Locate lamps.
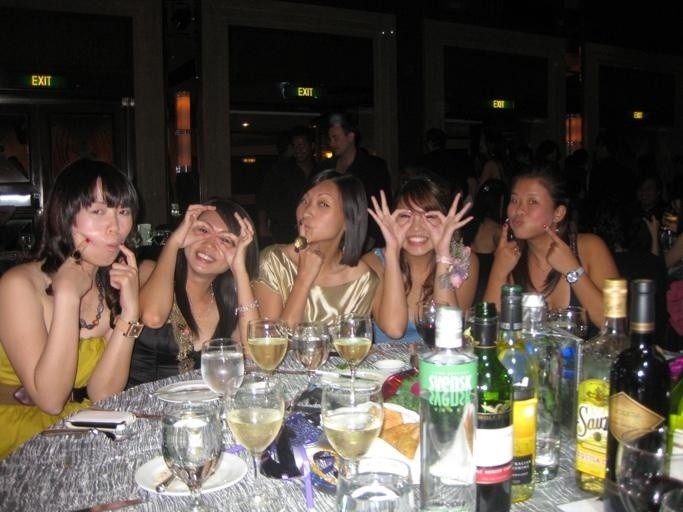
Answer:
[564,111,584,158]
[170,87,195,176]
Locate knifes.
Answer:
[67,498,144,511]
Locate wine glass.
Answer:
[200,312,377,405]
[158,376,415,512]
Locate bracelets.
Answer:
[234,299,260,316]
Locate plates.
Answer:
[372,359,405,374]
[155,379,226,405]
[135,452,248,497]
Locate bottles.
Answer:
[415,276,683,512]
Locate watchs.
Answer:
[112,316,143,339]
[564,268,586,288]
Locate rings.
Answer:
[293,236,306,253]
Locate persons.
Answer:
[252,166,380,341]
[125,195,261,391]
[481,166,625,340]
[359,173,481,342]
[0,158,141,461]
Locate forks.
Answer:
[38,429,144,442]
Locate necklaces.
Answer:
[166,284,216,325]
[78,272,105,331]
